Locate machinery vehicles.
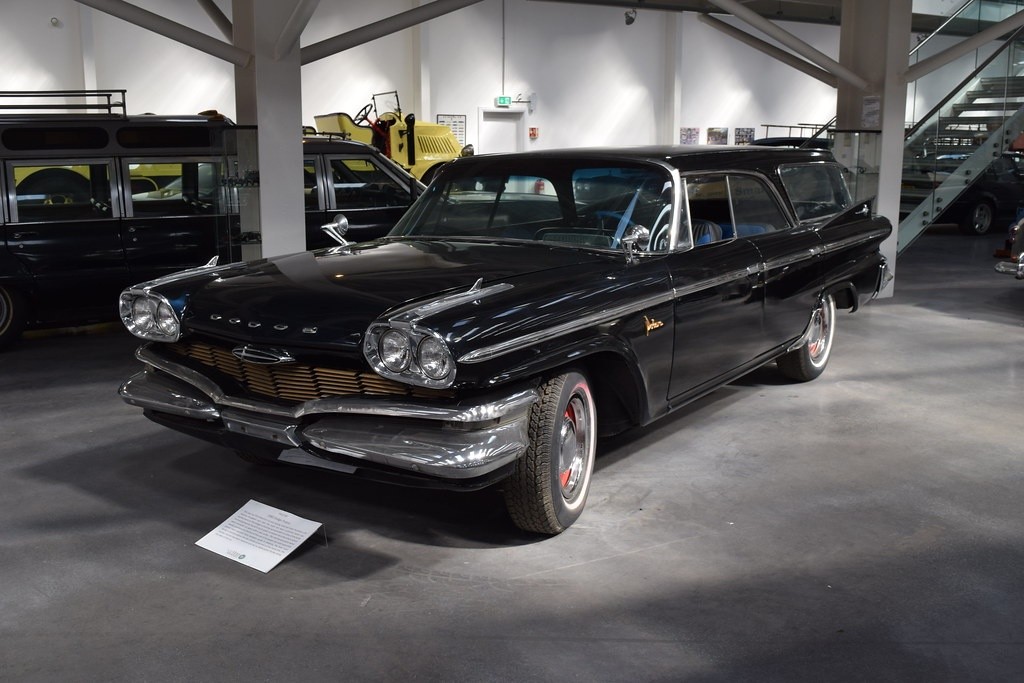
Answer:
[15,92,463,202]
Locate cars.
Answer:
[119,138,893,533]
[910,149,1024,237]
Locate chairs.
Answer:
[657,218,723,248]
[723,222,775,240]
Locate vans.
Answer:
[0,90,602,348]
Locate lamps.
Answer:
[624,8,638,25]
